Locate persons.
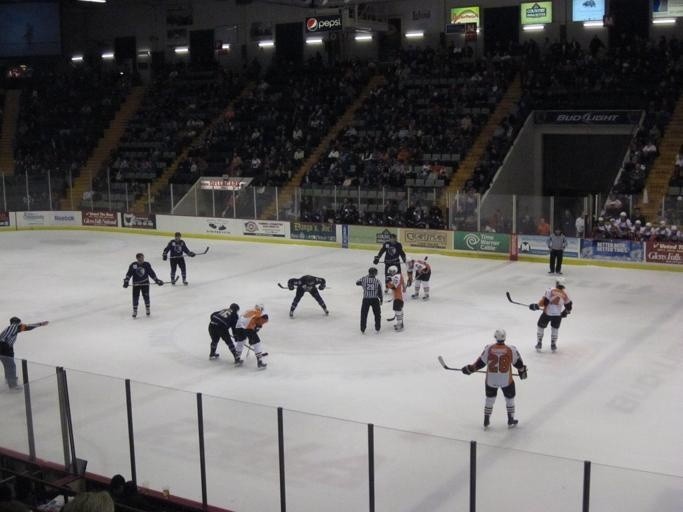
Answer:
[234,304,268,367]
[164,232,198,284]
[2,33,681,241]
[121,253,164,317]
[407,259,431,301]
[110,475,126,502]
[530,278,573,351]
[208,304,240,361]
[287,273,329,316]
[0,317,50,392]
[355,267,383,331]
[386,266,404,329]
[547,226,569,274]
[373,234,408,292]
[124,481,137,506]
[462,328,527,425]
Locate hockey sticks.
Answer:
[126,277,180,284]
[506,291,542,309]
[374,256,428,264]
[161,246,209,259]
[278,280,319,291]
[386,316,398,321]
[230,333,267,356]
[245,342,251,359]
[437,356,524,376]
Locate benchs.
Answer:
[1,457,147,512]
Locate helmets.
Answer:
[305,234,413,285]
[556,276,565,286]
[136,232,265,311]
[494,328,506,342]
[10,317,20,324]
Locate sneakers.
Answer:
[289,289,429,332]
[484,415,489,426]
[536,342,541,348]
[551,344,556,350]
[210,352,267,368]
[133,310,137,316]
[509,414,518,424]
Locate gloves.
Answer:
[518,365,528,379]
[561,311,567,317]
[123,281,129,287]
[462,364,475,374]
[530,304,539,311]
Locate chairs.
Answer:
[519,48,682,235]
